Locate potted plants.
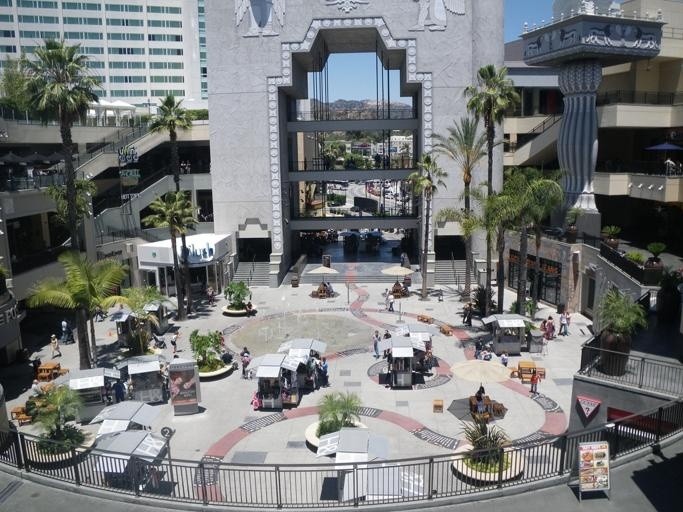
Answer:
[565,206,584,243]
[596,284,649,376]
[655,264,682,328]
[601,224,621,251]
[644,241,667,268]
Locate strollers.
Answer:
[479,345,491,360]
[152,331,167,350]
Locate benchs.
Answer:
[415,314,434,326]
[439,324,452,336]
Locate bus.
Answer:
[327,181,349,191]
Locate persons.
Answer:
[372,329,380,359]
[663,158,676,172]
[321,357,328,387]
[384,348,391,370]
[378,205,385,217]
[387,292,394,311]
[475,395,486,412]
[170,331,182,353]
[30,379,49,399]
[303,157,308,171]
[391,281,408,299]
[245,300,255,317]
[50,333,61,359]
[318,281,332,299]
[215,328,251,380]
[24,395,39,423]
[60,317,75,345]
[462,303,468,324]
[374,152,389,170]
[186,160,191,174]
[382,329,391,359]
[502,351,507,366]
[179,160,186,174]
[94,305,104,321]
[473,336,492,361]
[200,209,213,221]
[383,288,389,309]
[159,361,169,390]
[466,303,473,325]
[170,373,183,398]
[180,374,195,390]
[111,377,125,403]
[474,385,486,397]
[528,370,541,395]
[203,284,215,305]
[539,309,571,341]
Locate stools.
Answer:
[470,394,505,423]
[507,361,546,384]
[433,399,443,413]
[9,361,69,427]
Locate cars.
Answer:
[364,178,405,200]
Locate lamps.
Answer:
[627,182,663,193]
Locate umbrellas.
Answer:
[305,265,339,282]
[84,94,136,117]
[380,264,414,282]
[0,152,75,166]
[644,141,682,159]
